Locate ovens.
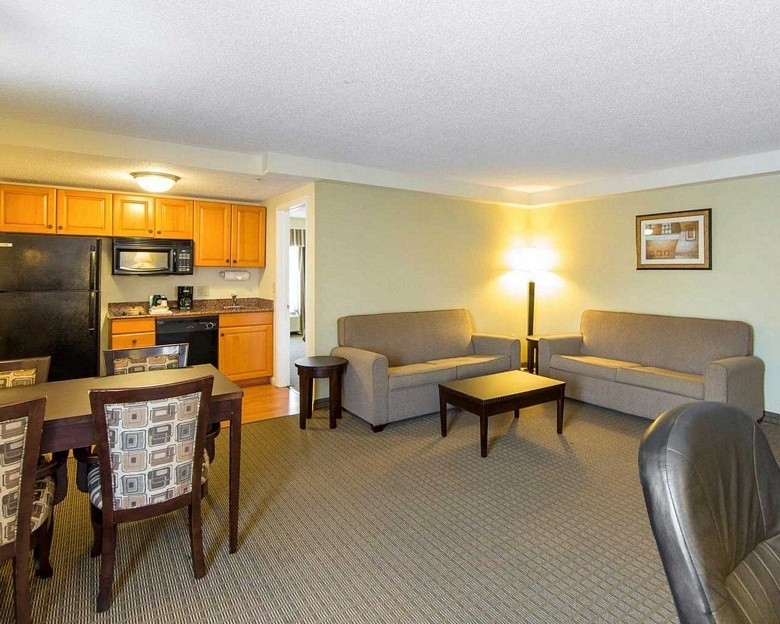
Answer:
[154,315,219,370]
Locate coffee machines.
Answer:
[177,286,193,309]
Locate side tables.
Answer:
[295,356,349,430]
[524,333,549,377]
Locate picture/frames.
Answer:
[635,207,713,271]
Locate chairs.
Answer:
[0,356,69,624]
[637,399,780,624]
[73,343,208,614]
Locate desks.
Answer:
[0,365,245,554]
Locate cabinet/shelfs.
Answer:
[0,184,112,237]
[111,317,155,349]
[113,194,193,239]
[219,312,272,379]
[195,201,266,267]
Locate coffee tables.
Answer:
[438,370,567,456]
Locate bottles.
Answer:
[160,296,168,306]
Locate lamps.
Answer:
[129,171,181,194]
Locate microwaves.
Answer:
[112,237,194,275]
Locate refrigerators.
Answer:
[0,232,102,383]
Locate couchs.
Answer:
[540,309,765,422]
[329,308,522,433]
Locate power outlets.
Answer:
[197,286,210,296]
